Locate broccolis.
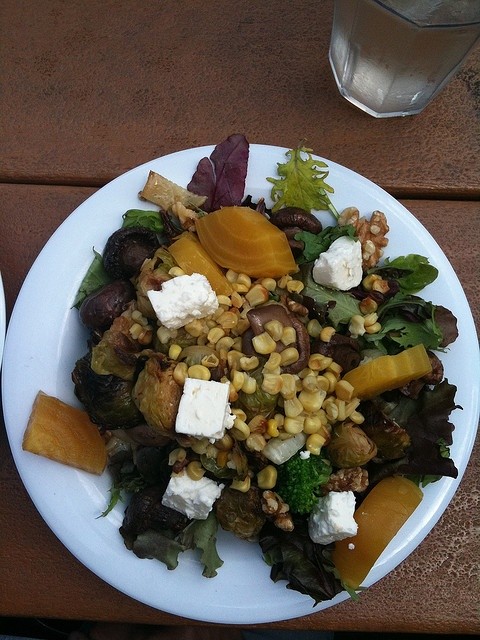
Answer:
[279,454,332,515]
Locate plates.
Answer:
[2,143,480,624]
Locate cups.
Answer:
[329,0,480,119]
